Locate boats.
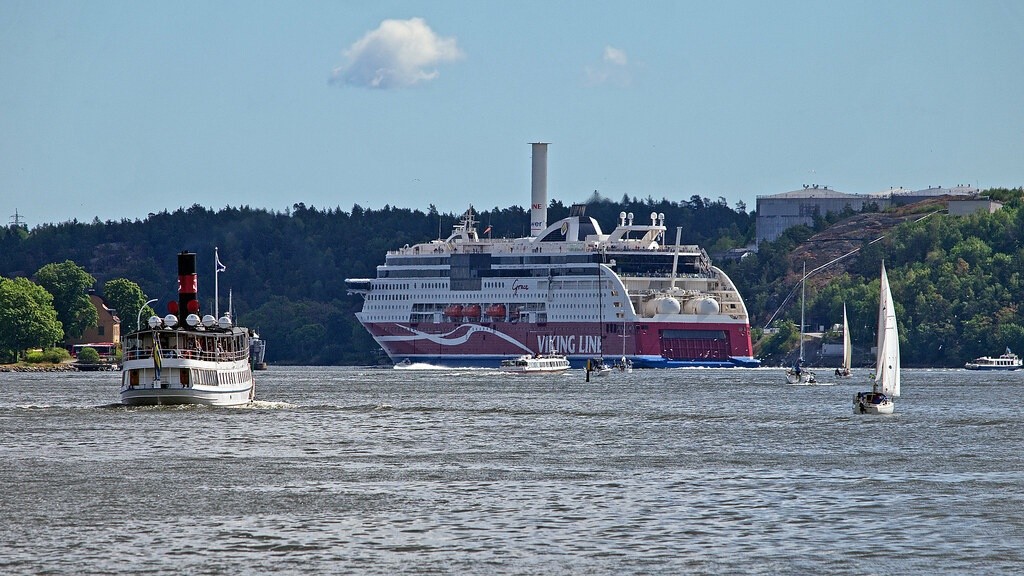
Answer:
[499,342,572,373]
[442,306,462,317]
[460,306,482,317]
[964,346,1023,371]
[121,247,257,406]
[345,143,761,370]
[210,301,267,371]
[485,306,505,316]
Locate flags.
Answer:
[150,329,162,381]
[215,251,226,272]
[483,228,491,234]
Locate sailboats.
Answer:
[785,261,816,384]
[835,302,854,379]
[853,259,900,414]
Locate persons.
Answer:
[129,338,222,361]
[842,369,849,376]
[534,353,540,359]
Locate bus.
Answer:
[72,342,116,358]
